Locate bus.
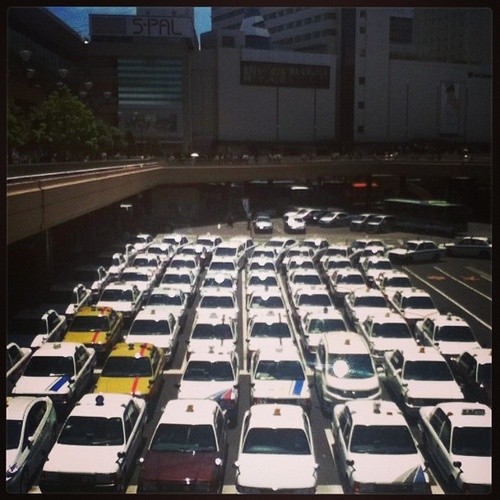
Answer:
[312,178,380,211]
[382,197,468,236]
[246,181,311,205]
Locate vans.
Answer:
[283,205,397,234]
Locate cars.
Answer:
[281,237,492,404]
[38,392,147,493]
[388,240,446,264]
[255,213,272,232]
[6,232,222,401]
[234,403,318,493]
[136,398,229,492]
[6,396,58,490]
[440,238,492,260]
[331,397,426,493]
[176,232,254,400]
[419,401,492,490]
[247,236,316,415]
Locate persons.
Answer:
[242,152,248,160]
[168,152,175,160]
[225,212,233,229]
[268,151,281,163]
